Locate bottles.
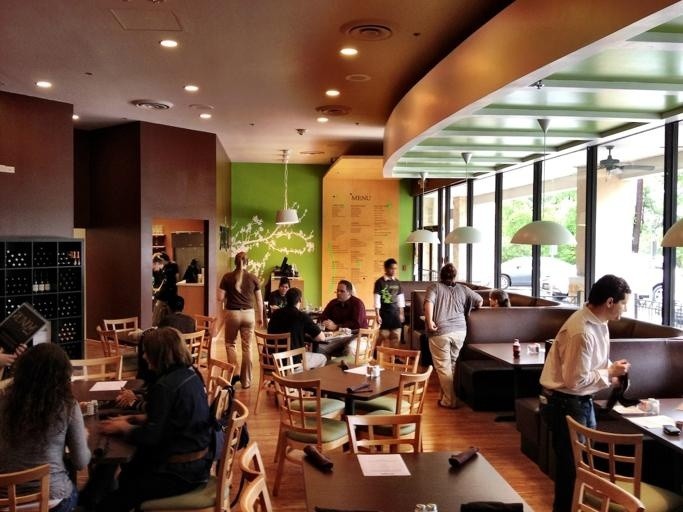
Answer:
[0,246,81,344]
[512,338,520,360]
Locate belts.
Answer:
[166,448,209,465]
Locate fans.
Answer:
[572,143,657,183]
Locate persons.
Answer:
[315,280,368,364]
[152,251,179,325]
[536,275,633,511]
[216,252,264,388]
[422,265,483,406]
[0,344,92,511]
[114,326,207,416]
[267,277,292,317]
[155,299,198,336]
[181,258,201,283]
[373,258,406,365]
[263,287,327,369]
[0,342,28,381]
[94,327,216,511]
[488,290,514,310]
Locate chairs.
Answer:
[566,412,681,511]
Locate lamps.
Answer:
[404,151,481,247]
[275,150,299,225]
[510,81,579,248]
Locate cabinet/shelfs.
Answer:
[1,234,86,356]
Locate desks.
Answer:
[300,450,534,511]
[176,280,203,315]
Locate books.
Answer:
[0,302,49,353]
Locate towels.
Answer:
[460,501,526,512]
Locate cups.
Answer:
[647,397,660,416]
[366,365,380,380]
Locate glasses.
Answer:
[337,289,346,293]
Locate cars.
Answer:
[498,255,577,292]
